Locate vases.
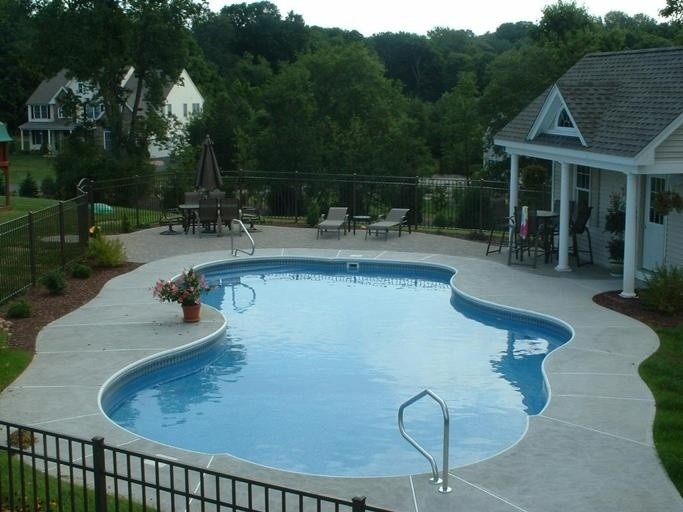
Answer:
[182,304,201,322]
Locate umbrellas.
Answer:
[192,134,222,199]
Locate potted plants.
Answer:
[606,239,625,278]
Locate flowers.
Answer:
[153,269,210,305]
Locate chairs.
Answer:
[485,199,594,269]
[315,206,349,240]
[366,207,412,240]
[155,192,264,236]
